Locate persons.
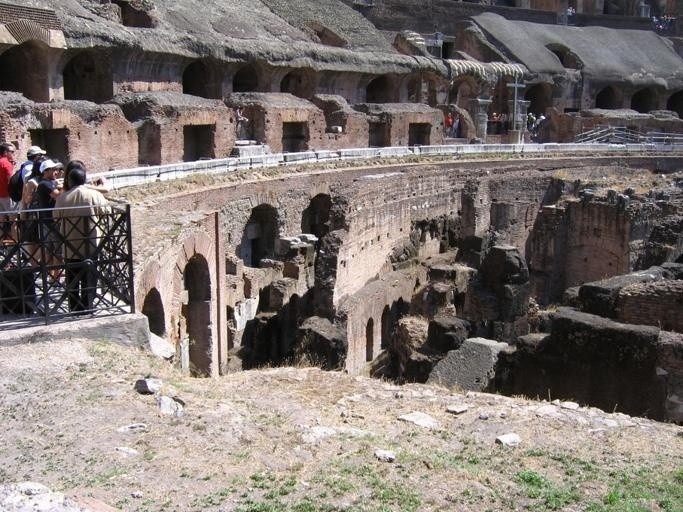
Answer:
[0,142,20,241]
[445,112,453,138]
[452,114,459,137]
[524,111,546,139]
[8,145,109,315]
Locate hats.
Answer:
[38,159,60,172]
[27,145,48,157]
